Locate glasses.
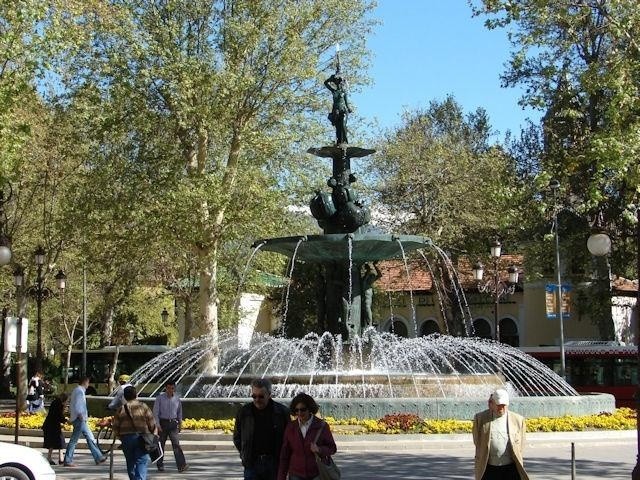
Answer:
[292,406,309,412]
[251,392,268,399]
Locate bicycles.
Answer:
[96,407,119,454]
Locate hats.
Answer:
[491,389,510,407]
[118,374,130,384]
[313,453,342,480]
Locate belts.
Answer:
[160,418,177,422]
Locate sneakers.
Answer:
[157,465,166,472]
[49,459,78,468]
[94,456,107,465]
[178,463,189,473]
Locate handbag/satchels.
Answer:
[137,432,159,454]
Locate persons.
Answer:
[25,369,190,480]
[323,73,352,144]
[471,388,530,480]
[233,377,292,479]
[277,393,337,479]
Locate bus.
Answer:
[504,339,639,413]
[59,344,201,398]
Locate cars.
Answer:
[0,439,57,479]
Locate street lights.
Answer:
[1,179,18,270]
[471,237,521,378]
[14,244,69,374]
[586,204,640,305]
